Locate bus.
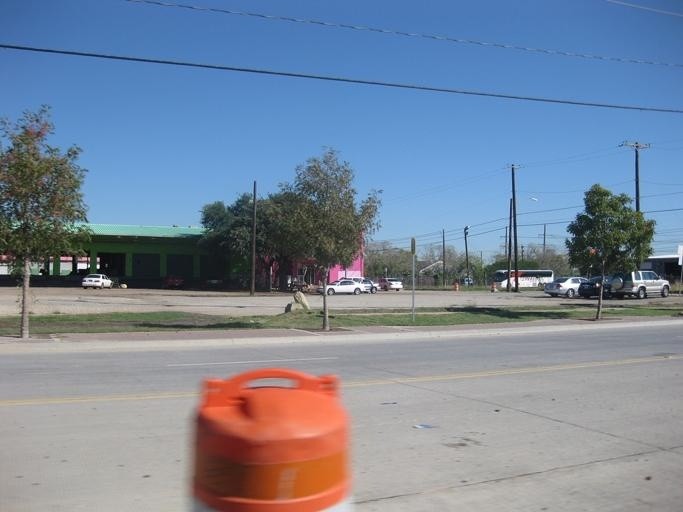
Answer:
[493,269,554,289]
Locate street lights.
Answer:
[520,245,524,263]
[505,196,539,292]
[463,225,470,288]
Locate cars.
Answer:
[356,278,376,294]
[375,278,403,291]
[339,277,364,284]
[202,275,223,291]
[287,281,309,290]
[545,277,589,299]
[81,274,113,290]
[316,280,365,295]
[578,275,626,299]
[160,275,184,290]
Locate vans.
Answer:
[452,277,473,288]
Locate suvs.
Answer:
[611,270,671,299]
